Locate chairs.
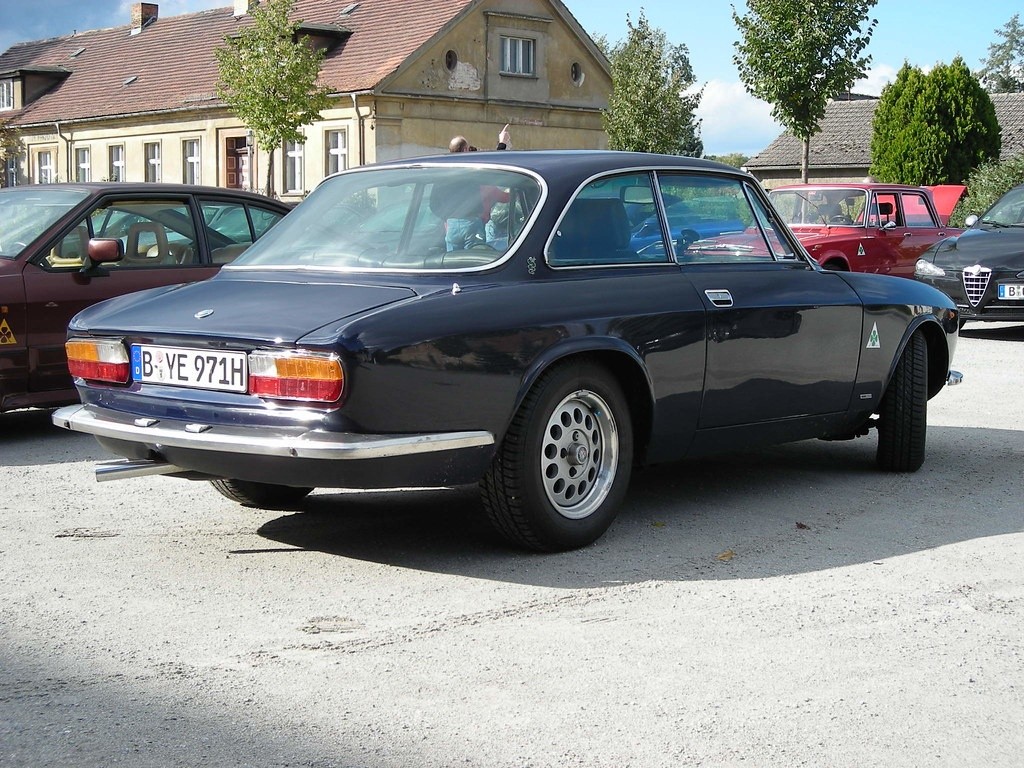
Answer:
[116,222,177,266]
[41,227,88,267]
[871,203,892,226]
[553,197,637,259]
[818,204,843,222]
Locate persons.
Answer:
[430,124,523,251]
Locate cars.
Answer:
[0,180,295,420]
[623,187,745,264]
[61,201,274,256]
[53,148,962,558]
[914,181,1024,332]
[687,181,968,284]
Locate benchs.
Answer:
[286,247,504,268]
[137,243,250,264]
